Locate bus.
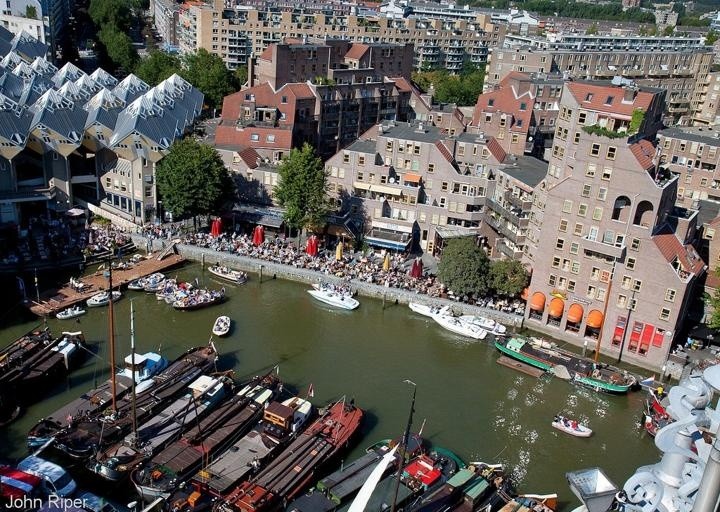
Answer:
[85,37,95,56]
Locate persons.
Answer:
[69,276,83,293]
[657,384,664,397]
[554,412,583,432]
[92,215,527,331]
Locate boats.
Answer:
[406,301,676,439]
[55,261,249,320]
[307,289,360,311]
[312,283,354,299]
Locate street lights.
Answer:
[614,289,640,365]
[157,200,163,238]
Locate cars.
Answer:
[144,9,162,42]
